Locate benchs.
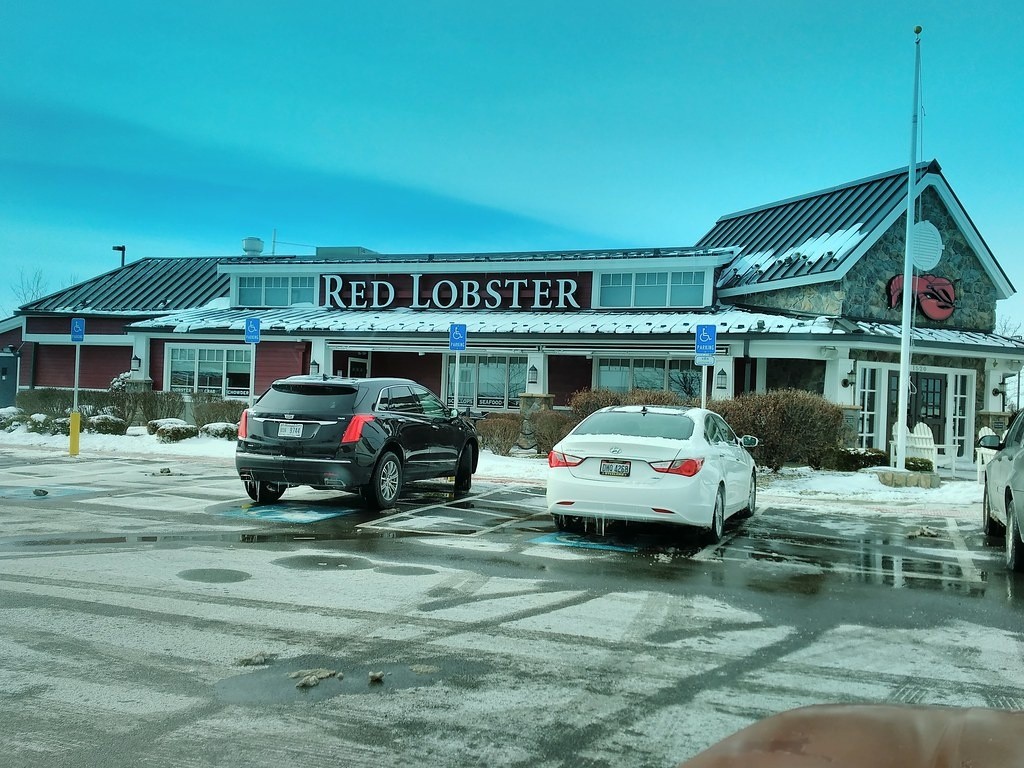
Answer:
[889,421,961,479]
[974,426,1010,483]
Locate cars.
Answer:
[235,373,479,509]
[546,405,758,543]
[977,407,1024,571]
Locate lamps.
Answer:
[130,355,142,371]
[716,368,727,389]
[310,360,319,375]
[2,346,17,353]
[528,364,538,383]
[847,370,856,386]
[993,377,1008,397]
